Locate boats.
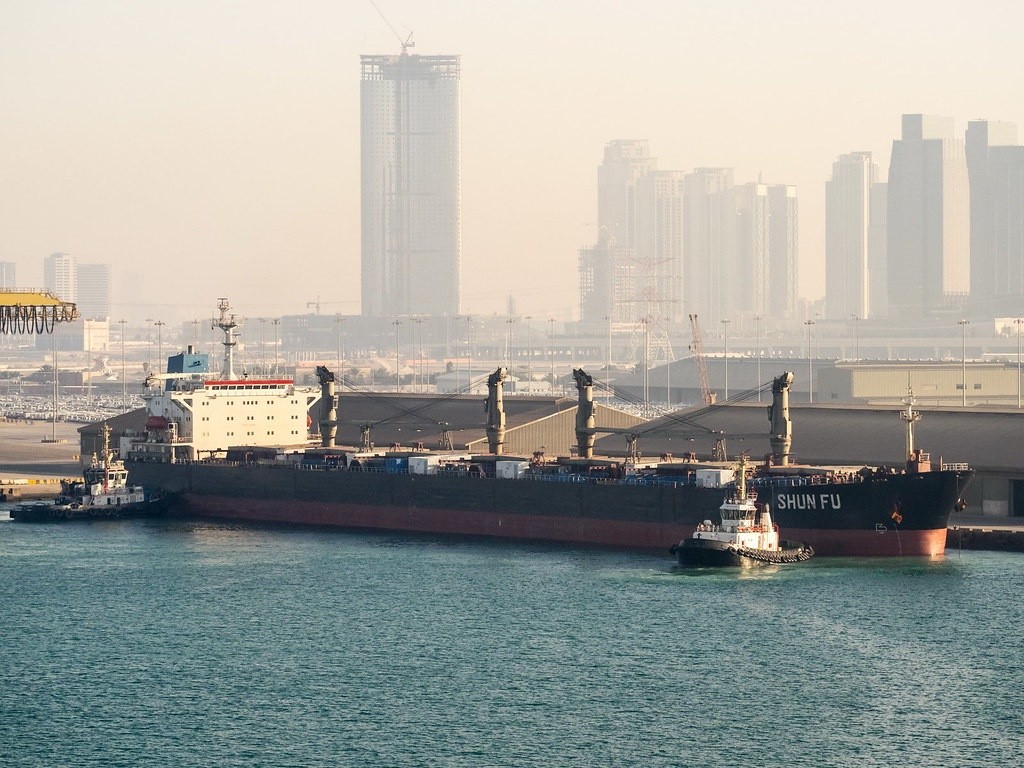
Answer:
[74,298,974,566]
[670,448,816,568]
[9,422,178,521]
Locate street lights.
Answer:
[547,318,556,397]
[849,312,861,365]
[153,320,166,395]
[146,319,153,376]
[602,317,610,391]
[119,319,127,414]
[753,314,763,403]
[410,319,417,395]
[464,317,473,394]
[416,319,425,394]
[392,320,402,394]
[639,317,652,414]
[334,317,347,391]
[524,314,534,396]
[957,320,971,406]
[663,314,673,408]
[244,316,281,374]
[191,320,200,355]
[506,318,516,396]
[803,319,816,403]
[1014,318,1024,409]
[720,318,732,401]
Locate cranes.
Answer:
[687,312,718,405]
[307,296,360,314]
